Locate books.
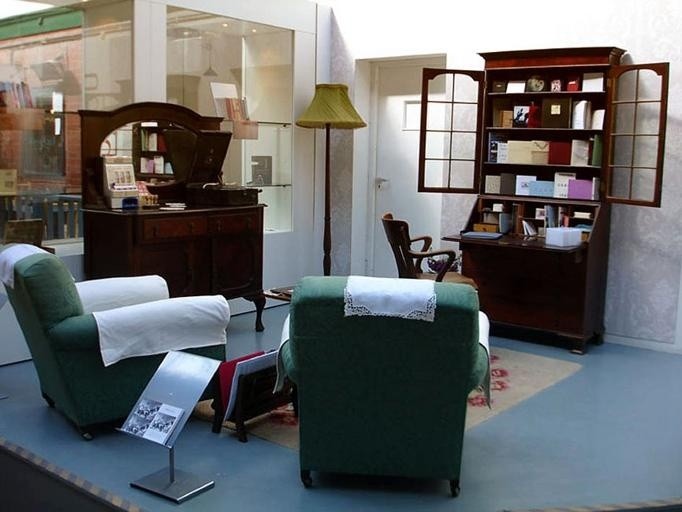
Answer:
[572,100,591,130]
[514,106,530,128]
[123,397,186,446]
[591,176,600,201]
[498,212,511,233]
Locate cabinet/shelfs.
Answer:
[216,119,295,234]
[442,45,627,354]
[78,198,268,331]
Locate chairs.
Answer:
[0,242,231,439]
[0,191,83,239]
[381,212,479,287]
[277,272,492,497]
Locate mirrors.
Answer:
[94,118,199,191]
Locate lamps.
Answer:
[296,81,368,275]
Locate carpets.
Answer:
[189,346,581,453]
